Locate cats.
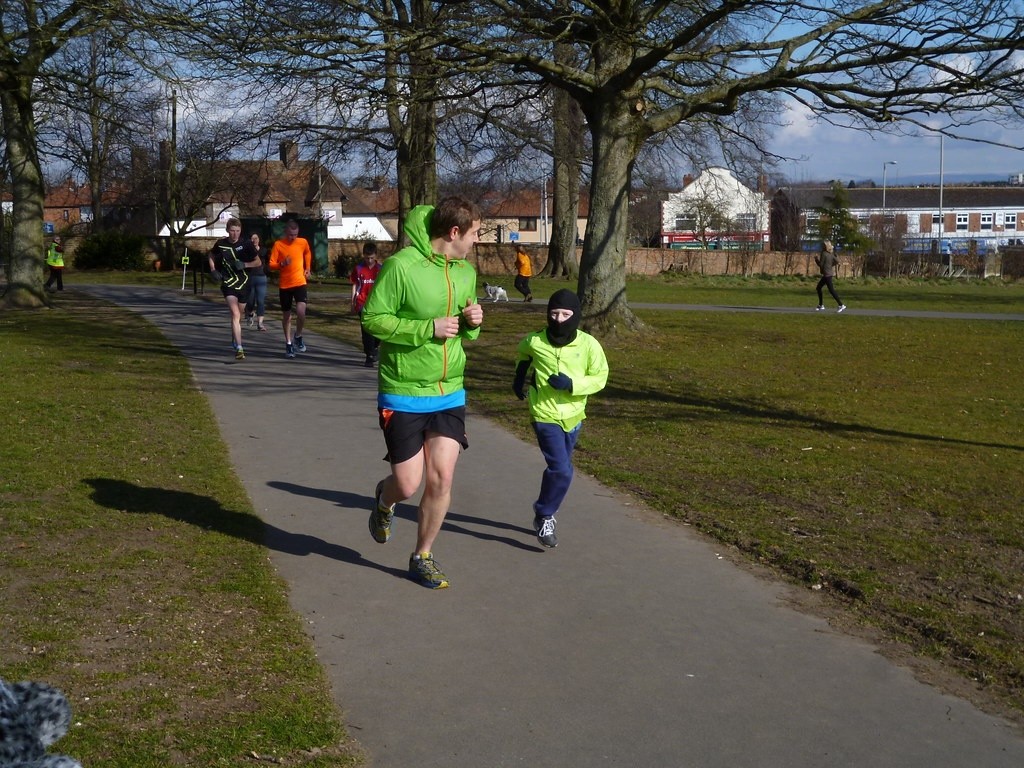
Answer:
[0,679,82,768]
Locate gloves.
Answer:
[512,383,526,401]
[548,372,572,393]
[212,270,222,281]
[235,261,245,270]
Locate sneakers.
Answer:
[232,338,243,349]
[370,480,396,543]
[235,348,245,359]
[373,348,378,362]
[533,503,559,548]
[286,343,296,358]
[294,333,306,352]
[408,552,451,589]
[365,355,373,367]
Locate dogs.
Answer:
[481,281,508,302]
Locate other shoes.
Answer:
[44,284,50,291]
[816,304,825,311]
[524,294,532,302]
[837,304,847,312]
[247,313,255,327]
[245,307,248,319]
[257,325,266,331]
[61,289,66,291]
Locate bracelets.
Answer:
[280,262,285,267]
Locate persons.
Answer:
[269,220,311,360]
[208,218,262,360]
[513,288,609,549]
[814,240,846,313]
[44,235,69,293]
[513,245,533,302]
[349,242,382,368]
[244,232,268,330]
[361,196,484,589]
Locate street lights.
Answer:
[544,172,554,246]
[920,133,944,254]
[760,153,772,251]
[882,160,897,250]
